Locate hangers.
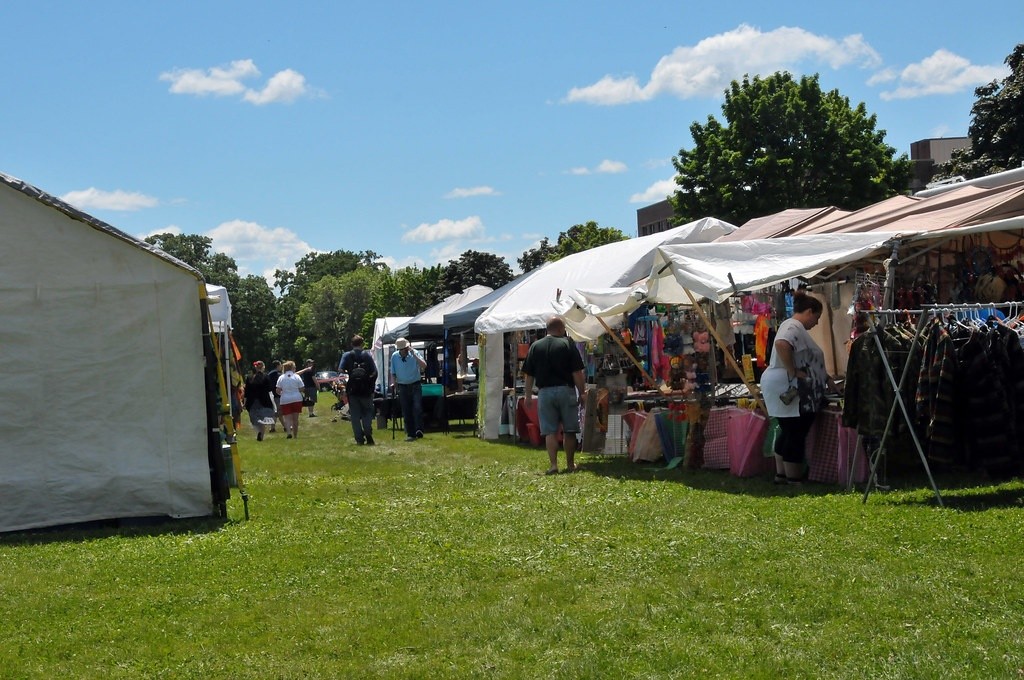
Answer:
[856,273,880,290]
[861,301,1024,345]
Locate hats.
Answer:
[306,359,314,363]
[394,337,411,350]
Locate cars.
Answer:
[313,371,346,391]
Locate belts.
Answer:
[539,383,572,388]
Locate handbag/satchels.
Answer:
[780,370,799,405]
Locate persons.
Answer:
[521,319,587,476]
[244,360,275,441]
[302,359,320,416]
[949,272,1006,321]
[275,360,305,438]
[338,334,378,445]
[759,296,828,483]
[267,360,287,432]
[390,336,428,441]
[234,381,244,431]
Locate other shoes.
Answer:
[286,433,292,438]
[284,428,287,432]
[546,467,577,475]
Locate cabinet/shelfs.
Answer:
[908,246,967,300]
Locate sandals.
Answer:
[774,473,812,486]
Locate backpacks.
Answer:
[346,352,370,399]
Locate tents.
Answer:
[474,165,1024,440]
[407,284,495,436]
[376,316,413,398]
[442,263,543,436]
[0,172,223,534]
[204,282,233,436]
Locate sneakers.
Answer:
[269,429,275,433]
[256,432,262,441]
[405,430,424,441]
[355,440,375,445]
[309,414,317,417]
